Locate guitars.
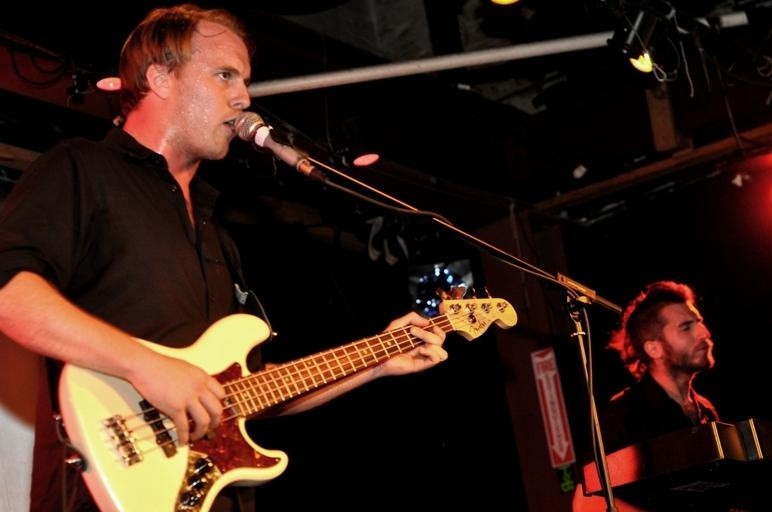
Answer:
[55,294,520,511]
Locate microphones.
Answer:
[235,113,327,181]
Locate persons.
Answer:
[604,279,772,510]
[0,3,451,510]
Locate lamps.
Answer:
[604,0,762,86]
[65,56,122,106]
[324,128,379,171]
